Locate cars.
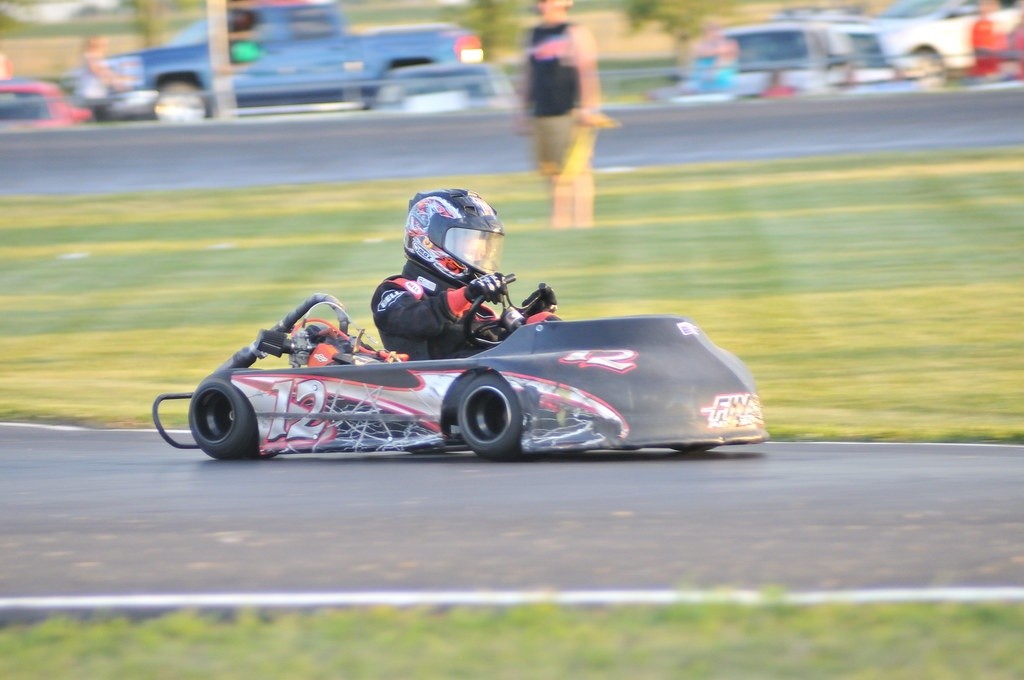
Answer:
[665,0,1024,101]
[0,75,93,131]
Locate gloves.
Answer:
[464,272,508,305]
[522,283,558,318]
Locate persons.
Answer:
[518,0,604,228]
[74,37,118,99]
[972,0,1024,84]
[686,17,739,93]
[370,188,557,360]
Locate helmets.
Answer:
[403,189,505,289]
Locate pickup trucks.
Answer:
[67,0,491,125]
